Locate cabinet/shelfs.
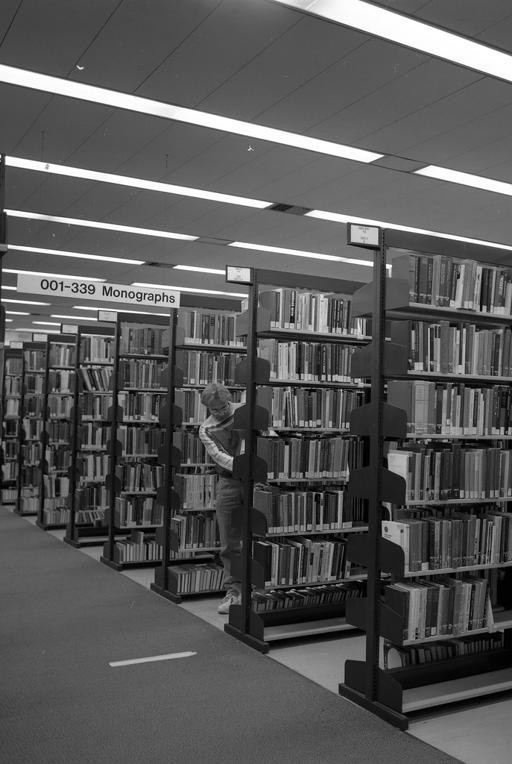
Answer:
[1,344,25,505]
[14,337,53,517]
[145,292,249,603]
[37,329,79,532]
[218,264,372,654]
[100,310,172,571]
[63,322,116,548]
[333,226,510,732]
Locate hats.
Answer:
[198,379,232,407]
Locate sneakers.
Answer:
[217,592,242,613]
